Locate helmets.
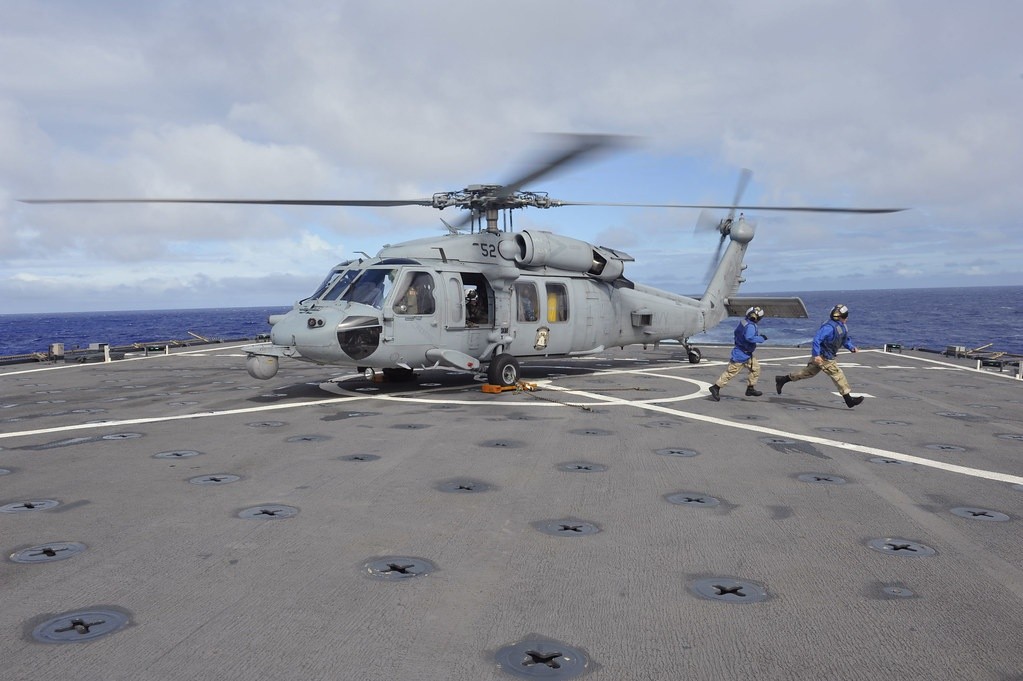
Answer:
[830,304,848,321]
[746,306,765,323]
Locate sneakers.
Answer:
[709,386,720,401]
[746,389,762,396]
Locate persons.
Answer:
[709,306,768,401]
[776,304,864,407]
[401,285,430,313]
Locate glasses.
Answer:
[840,314,847,318]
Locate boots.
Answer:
[776,375,791,394]
[843,393,863,408]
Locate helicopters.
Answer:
[14,131,910,387]
